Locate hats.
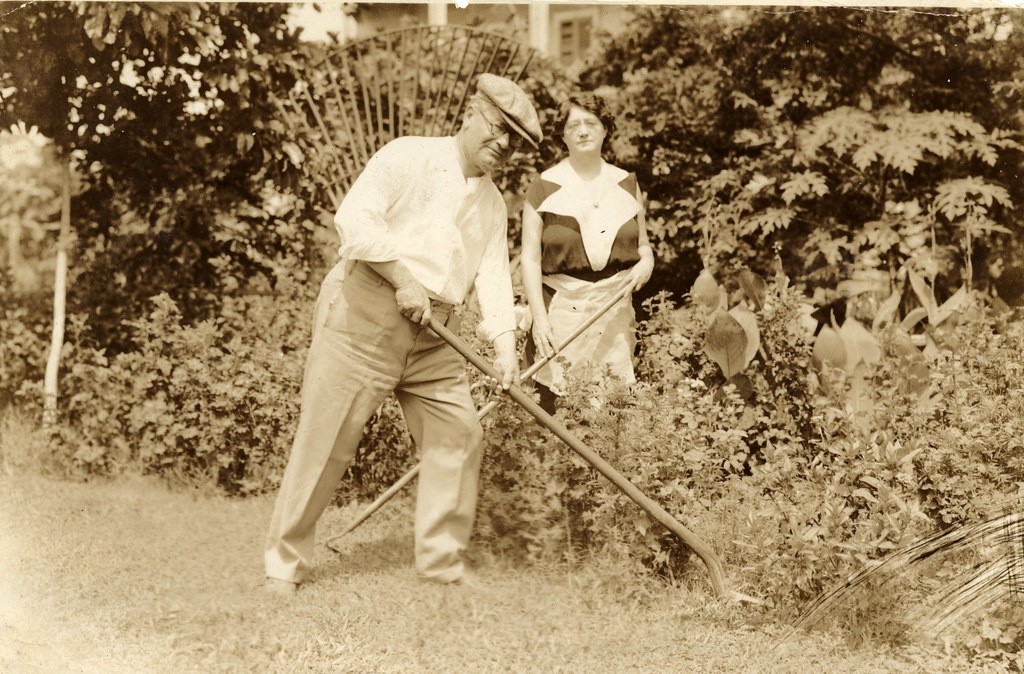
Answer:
[475,73,543,150]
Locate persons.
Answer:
[263,71,548,601]
[516,94,659,427]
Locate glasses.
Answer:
[475,107,524,148]
